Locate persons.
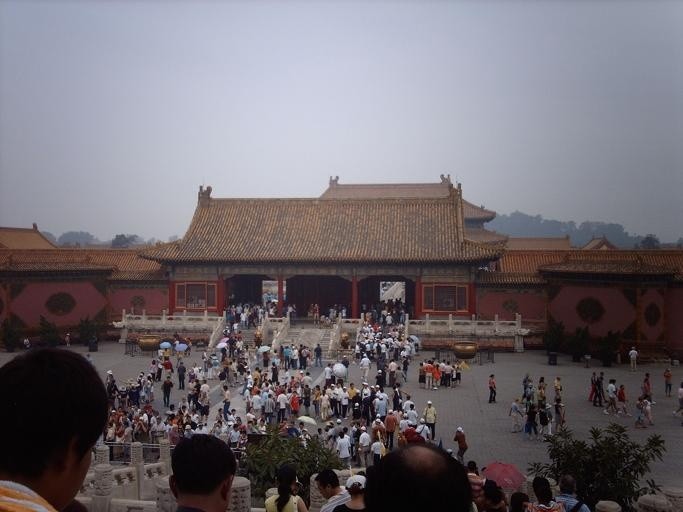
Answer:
[0,347,110,512]
[19,296,683,512]
